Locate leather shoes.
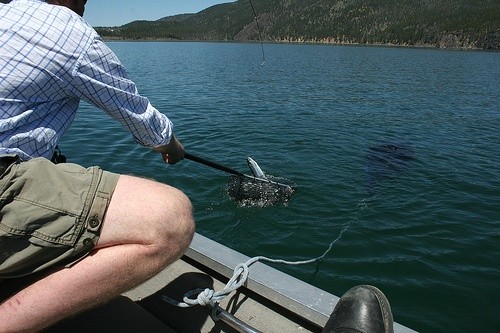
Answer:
[322,283,395,332]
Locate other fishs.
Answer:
[247,156,267,179]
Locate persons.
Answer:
[321,285,394,333]
[0,0,196,333]
